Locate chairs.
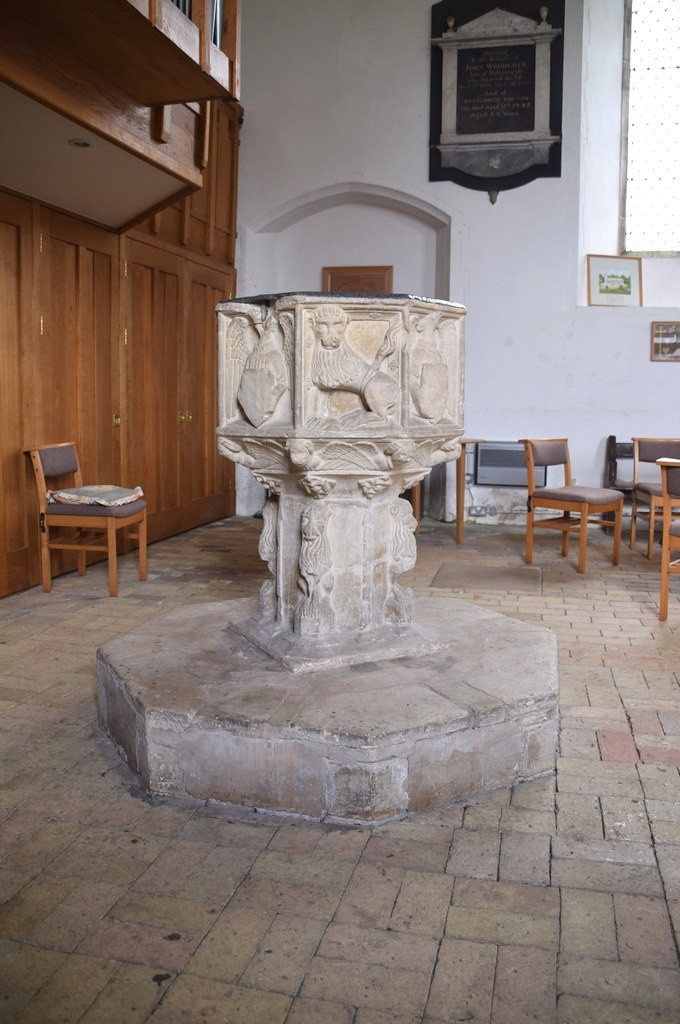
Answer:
[25,443,148,596]
[519,433,680,621]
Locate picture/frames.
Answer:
[588,253,644,305]
[651,320,680,361]
[322,265,393,294]
